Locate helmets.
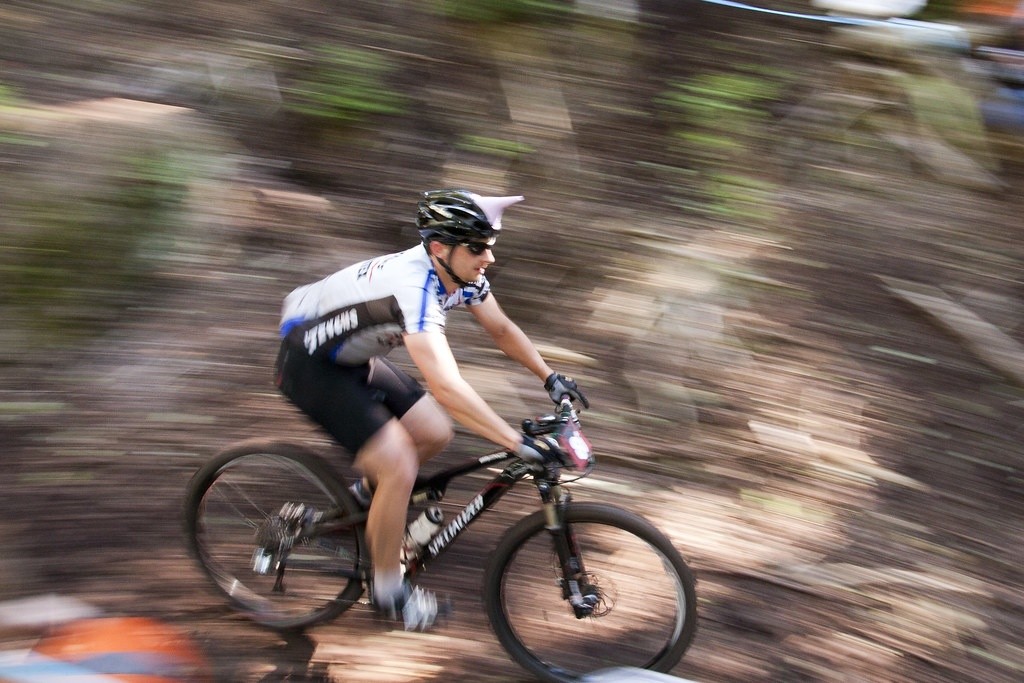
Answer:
[415,188,526,247]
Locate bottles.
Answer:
[400,507,443,566]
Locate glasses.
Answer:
[458,240,494,255]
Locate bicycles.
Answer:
[176,392,698,683]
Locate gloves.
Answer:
[543,370,590,410]
[513,433,562,464]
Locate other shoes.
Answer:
[347,481,372,507]
[372,579,453,634]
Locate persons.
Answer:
[272,189,590,630]
